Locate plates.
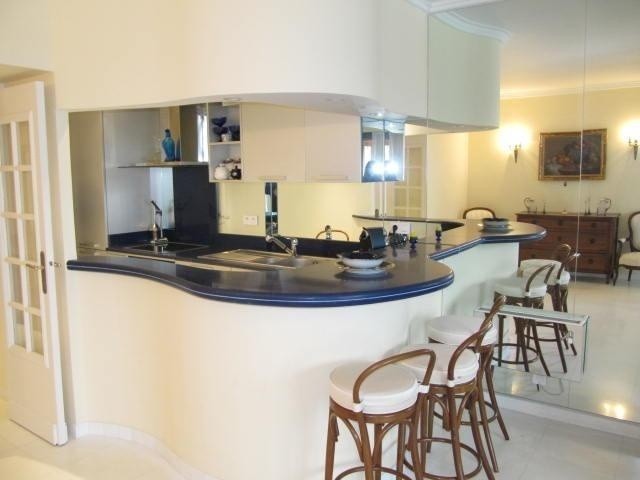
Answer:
[334,261,395,274]
[478,224,512,229]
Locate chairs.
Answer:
[402,320,496,480]
[429,293,513,441]
[478,239,589,387]
[463,203,492,220]
[614,209,640,284]
[326,349,436,480]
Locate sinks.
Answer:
[247,255,326,271]
[125,241,210,256]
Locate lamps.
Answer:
[505,125,526,163]
[383,160,400,181]
[622,116,640,160]
[364,160,383,182]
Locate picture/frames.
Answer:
[538,127,609,183]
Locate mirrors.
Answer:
[427,7,640,418]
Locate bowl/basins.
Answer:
[336,252,389,268]
[482,218,509,226]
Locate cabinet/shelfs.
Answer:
[306,108,363,184]
[208,101,241,182]
[241,104,307,183]
[514,209,621,286]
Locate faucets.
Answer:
[150,199,168,243]
[265,234,298,257]
